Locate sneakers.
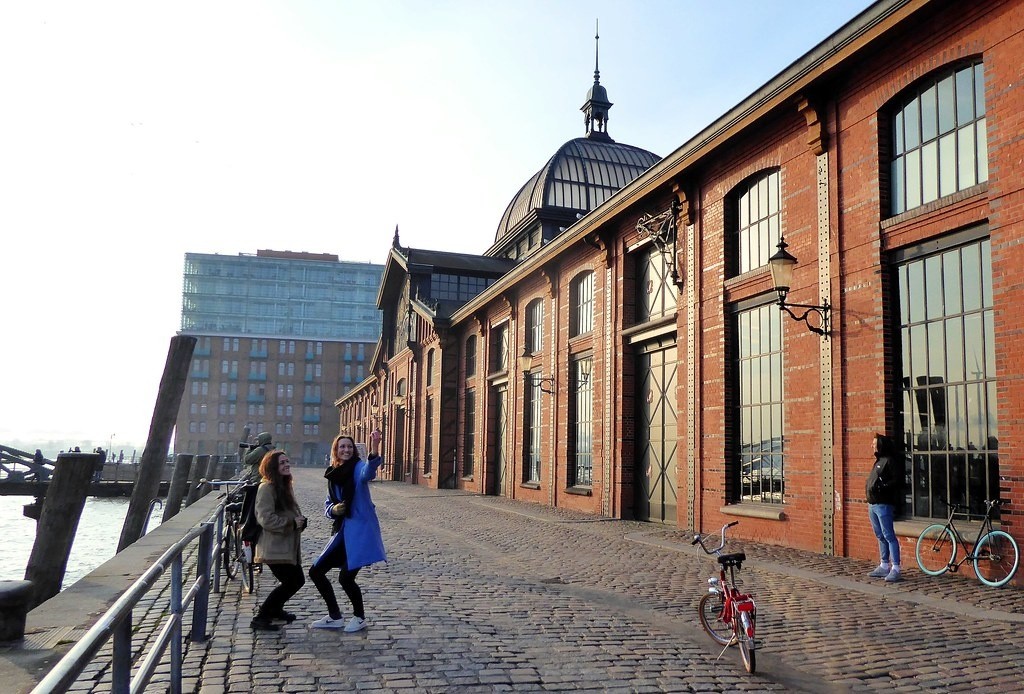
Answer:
[312,615,344,627]
[885,569,901,581]
[344,616,366,632]
[867,566,889,576]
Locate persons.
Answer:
[34,446,106,483]
[866,433,902,582]
[243,432,276,484]
[309,431,386,633]
[250,450,305,630]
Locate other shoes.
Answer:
[273,611,296,621]
[251,623,279,631]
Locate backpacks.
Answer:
[239,482,263,543]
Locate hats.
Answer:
[254,437,259,440]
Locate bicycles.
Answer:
[194,476,264,593]
[692,517,761,675]
[913,498,1020,588]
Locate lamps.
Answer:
[518,347,556,396]
[393,389,414,419]
[766,233,831,340]
[371,400,386,424]
